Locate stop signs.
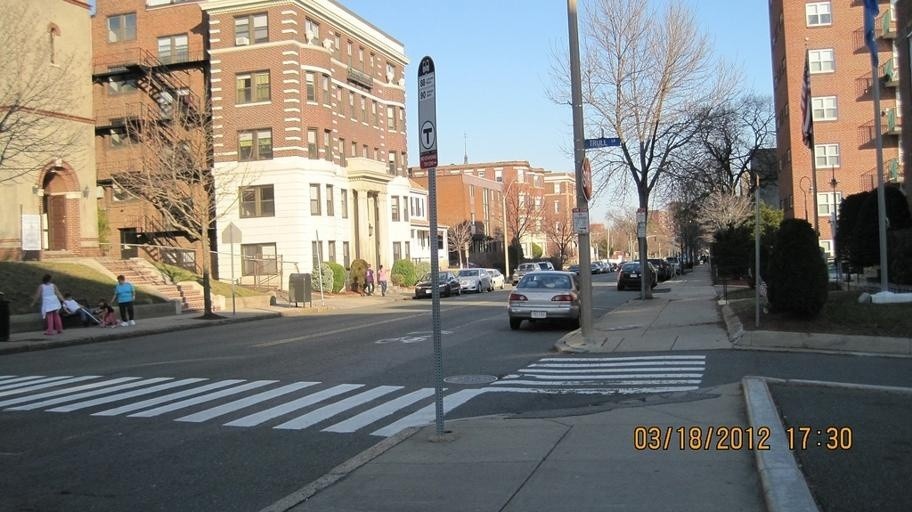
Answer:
[580,157,593,202]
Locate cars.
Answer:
[411,253,692,330]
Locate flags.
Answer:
[862,0,879,70]
[799,50,814,151]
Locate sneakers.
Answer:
[129,319,136,326]
[120,321,128,327]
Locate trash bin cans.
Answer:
[0,292,12,342]
[288,274,312,308]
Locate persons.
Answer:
[378,264,388,297]
[110,275,137,328]
[62,292,101,327]
[30,274,68,336]
[366,264,375,296]
[96,298,118,328]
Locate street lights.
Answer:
[502,171,535,283]
